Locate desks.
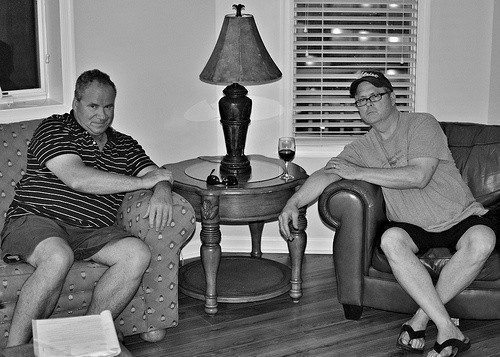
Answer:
[161,154,310,318]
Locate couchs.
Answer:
[0,117,196,343]
[319,121,500,321]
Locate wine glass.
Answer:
[278,137,296,180]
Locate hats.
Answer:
[350,71,393,95]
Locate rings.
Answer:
[334,164,336,168]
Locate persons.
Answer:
[278,71,500,357]
[0,69,174,347]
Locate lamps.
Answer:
[199,3,282,178]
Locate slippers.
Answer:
[433,336,472,357]
[396,321,427,354]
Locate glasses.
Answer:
[355,89,393,108]
